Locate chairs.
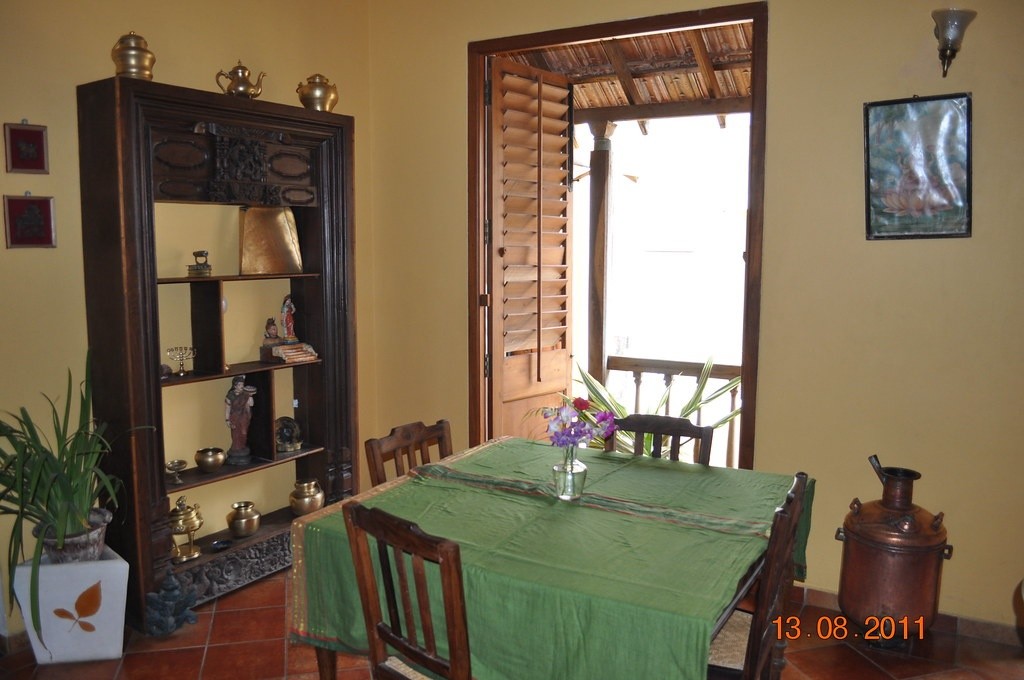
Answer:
[707,471,809,680]
[363,420,454,488]
[341,500,473,680]
[604,413,713,465]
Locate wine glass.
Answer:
[166,460,187,484]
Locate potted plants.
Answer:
[0,345,159,643]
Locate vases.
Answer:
[553,445,588,503]
[10,542,131,666]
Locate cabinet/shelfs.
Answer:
[75,76,361,636]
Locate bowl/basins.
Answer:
[194,447,226,472]
[212,539,233,550]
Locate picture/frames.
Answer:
[2,123,50,174]
[863,91,973,239]
[2,193,59,250]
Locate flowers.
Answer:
[542,392,615,466]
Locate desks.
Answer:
[284,432,816,680]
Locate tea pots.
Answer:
[215,60,266,99]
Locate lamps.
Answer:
[931,6,978,78]
